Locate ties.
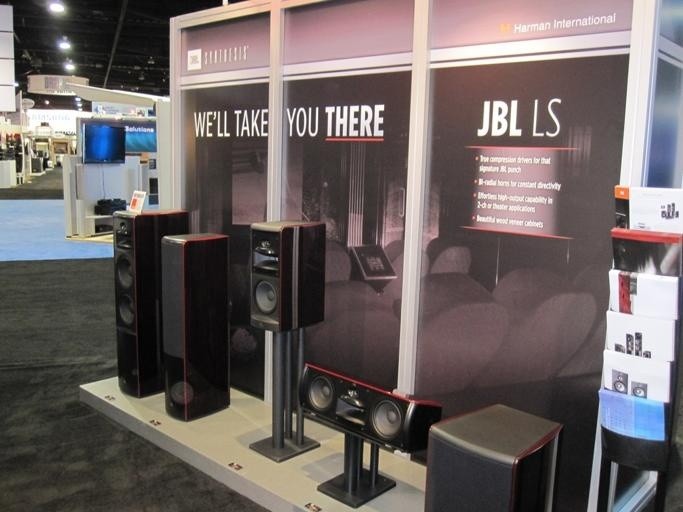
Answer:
[124,120,157,153]
[82,123,125,164]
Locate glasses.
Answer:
[59,35,71,50]
[65,58,75,70]
[49,0,65,13]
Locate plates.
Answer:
[597,270,683,512]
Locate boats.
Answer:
[112,210,189,398]
[249,220,326,463]
[298,361,443,508]
[161,232,230,422]
[423,403,564,511]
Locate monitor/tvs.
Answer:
[598,185,683,441]
